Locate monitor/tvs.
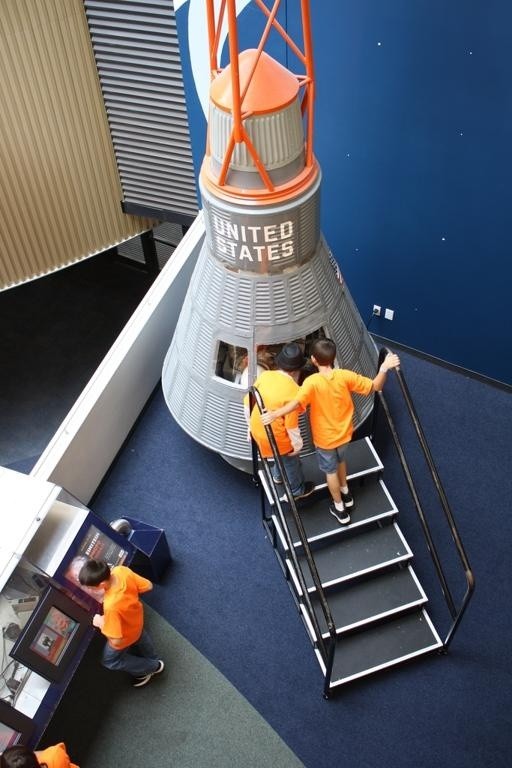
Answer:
[8,582,91,685]
[52,509,130,615]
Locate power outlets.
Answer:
[373,305,381,316]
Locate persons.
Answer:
[69,556,114,603]
[242,342,316,503]
[0,742,81,768]
[78,559,165,689]
[259,338,401,525]
[236,345,275,389]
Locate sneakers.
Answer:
[283,481,315,503]
[132,660,164,687]
[329,491,354,525]
[272,476,282,484]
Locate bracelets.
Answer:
[378,367,388,373]
